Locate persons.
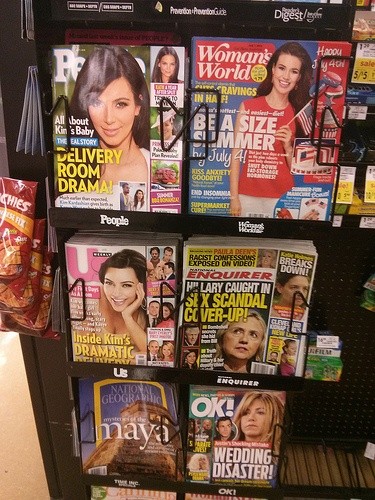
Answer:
[268,351,279,363]
[160,262,176,280]
[75,249,147,354]
[162,247,174,263]
[147,340,160,361]
[132,189,146,211]
[147,247,164,281]
[188,418,201,435]
[184,327,200,346]
[229,42,314,218]
[256,249,276,269]
[186,453,211,481]
[160,340,175,361]
[146,300,160,328]
[202,420,213,441]
[213,416,235,441]
[67,46,151,191]
[156,302,175,328]
[182,349,199,369]
[120,182,132,211]
[231,391,285,476]
[270,268,309,319]
[151,46,184,83]
[151,108,182,142]
[213,308,266,373]
[280,339,297,376]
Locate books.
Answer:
[64,229,318,378]
[52,37,352,221]
[80,378,286,488]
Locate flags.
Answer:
[296,100,319,136]
